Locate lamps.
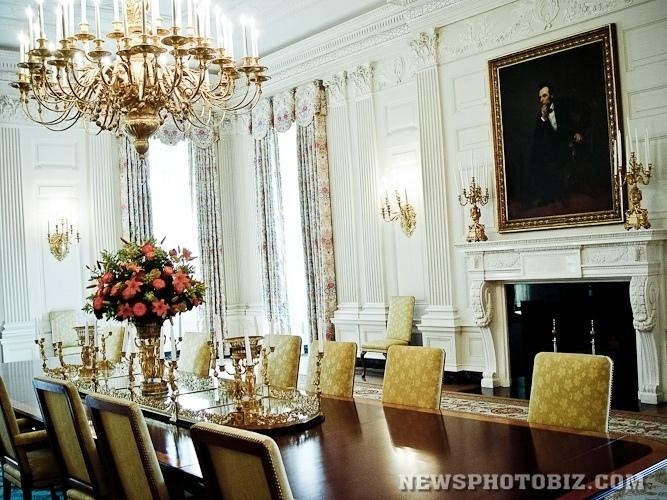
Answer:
[8,0,270,163]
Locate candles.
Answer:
[452,148,490,197]
[47,213,79,238]
[378,185,410,211]
[612,118,655,179]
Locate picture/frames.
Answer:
[487,23,631,234]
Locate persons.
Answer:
[530,82,590,208]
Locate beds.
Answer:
[0,354,667,499]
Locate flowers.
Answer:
[80,231,210,325]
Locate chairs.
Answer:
[46,310,446,413]
[189,420,293,499]
[0,375,83,499]
[527,351,616,433]
[33,378,103,500]
[360,295,416,380]
[85,392,205,498]
